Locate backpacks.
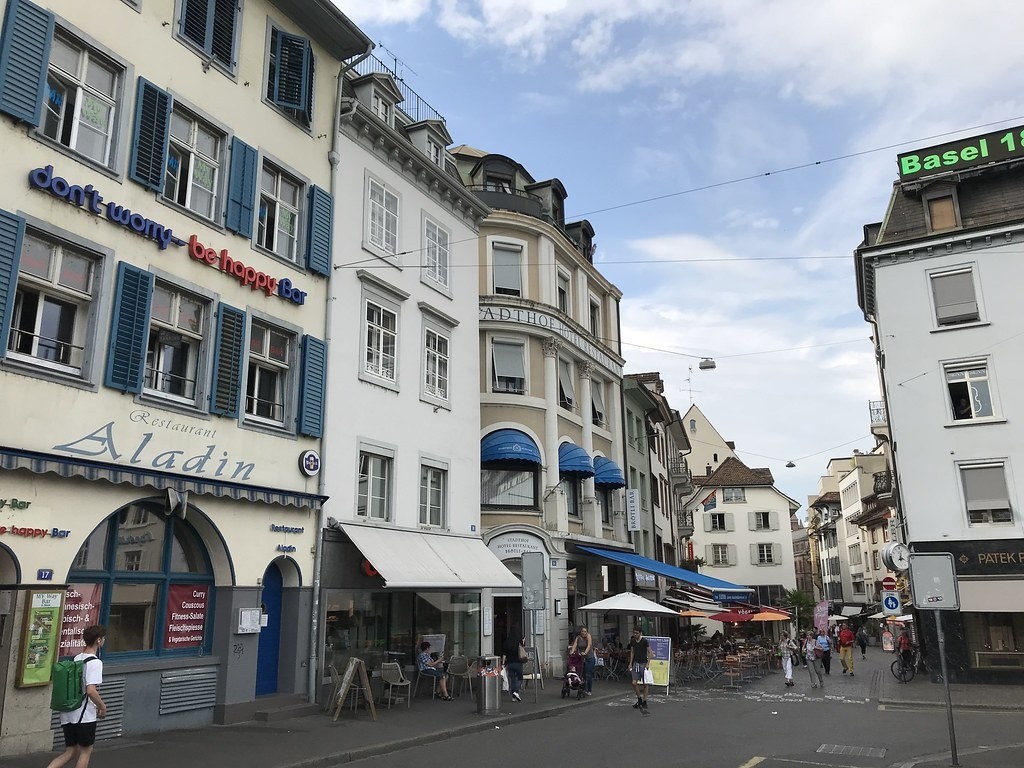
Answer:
[50,656,98,712]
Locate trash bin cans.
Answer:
[477,653,502,715]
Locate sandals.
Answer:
[436,692,443,699]
[444,696,455,701]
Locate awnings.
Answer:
[576,546,755,600]
[481,430,626,490]
[329,517,521,588]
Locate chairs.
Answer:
[446,655,471,697]
[451,661,478,700]
[324,664,358,714]
[379,662,412,710]
[413,658,449,700]
[593,639,775,692]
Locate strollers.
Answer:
[561,652,586,700]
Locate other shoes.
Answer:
[850,672,854,676]
[820,681,825,687]
[862,656,866,660]
[843,667,849,674]
[512,698,518,702]
[513,692,522,701]
[632,700,647,709]
[588,692,591,696]
[811,683,817,688]
[785,682,795,686]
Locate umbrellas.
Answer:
[577,592,682,617]
[680,610,791,641]
[828,612,913,631]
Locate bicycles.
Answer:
[891,646,915,684]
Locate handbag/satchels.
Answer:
[500,666,509,691]
[814,641,823,658]
[518,643,529,664]
[644,667,653,685]
[790,654,799,667]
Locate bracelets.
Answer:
[502,664,505,665]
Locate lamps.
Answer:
[786,461,796,468]
[698,358,717,372]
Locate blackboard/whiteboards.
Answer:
[522,647,542,679]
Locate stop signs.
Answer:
[882,577,896,590]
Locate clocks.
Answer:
[890,545,910,569]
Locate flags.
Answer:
[701,490,717,512]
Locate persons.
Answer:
[803,631,824,688]
[709,630,761,656]
[797,622,868,676]
[417,642,455,700]
[602,634,623,650]
[924,635,935,655]
[502,624,525,702]
[567,664,581,687]
[571,626,594,695]
[779,630,797,686]
[628,626,652,708]
[898,629,910,671]
[46,625,106,768]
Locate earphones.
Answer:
[98,641,100,643]
